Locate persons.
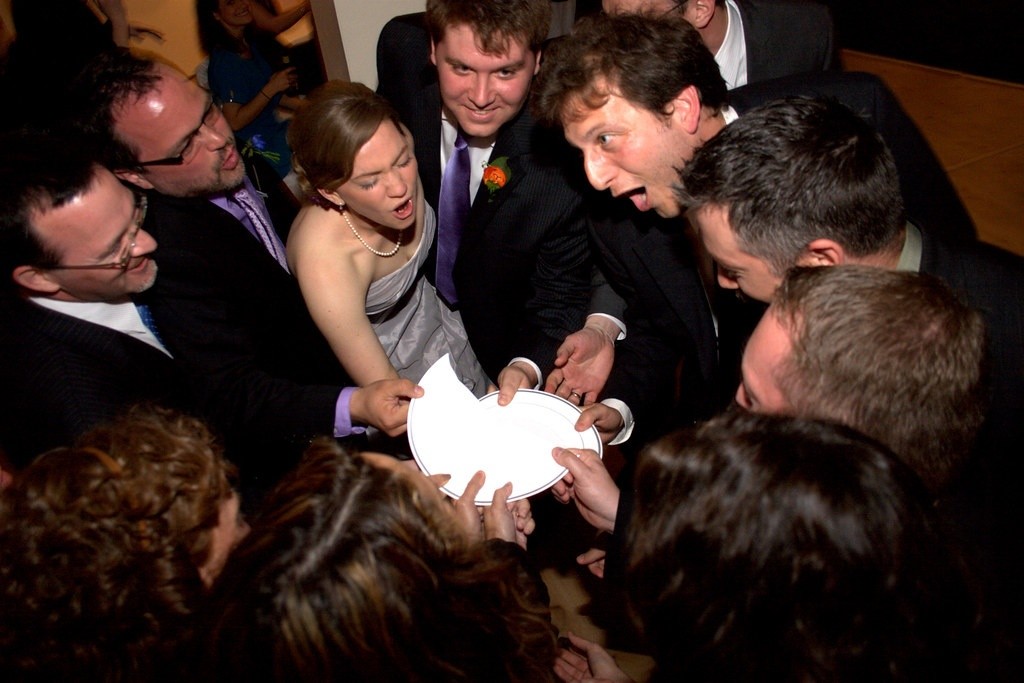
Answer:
[0,0,1024,683]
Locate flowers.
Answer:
[481,155,513,195]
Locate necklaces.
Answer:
[340,207,402,256]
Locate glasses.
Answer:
[35,190,148,270]
[124,86,223,167]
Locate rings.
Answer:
[571,392,581,400]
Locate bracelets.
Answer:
[260,90,271,101]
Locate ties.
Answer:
[435,123,475,306]
[231,187,291,279]
[130,292,168,349]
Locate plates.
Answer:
[407,352,603,504]
